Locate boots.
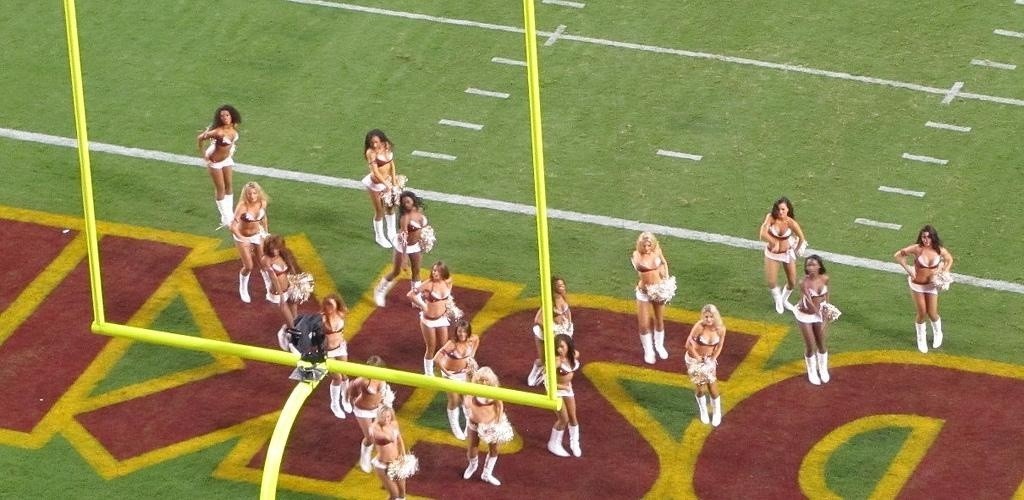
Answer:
[447,406,466,441]
[481,453,501,486]
[915,321,929,354]
[816,349,830,383]
[639,332,656,365]
[373,276,394,308]
[373,217,393,249]
[770,287,785,314]
[330,382,347,419]
[695,395,710,424]
[260,270,274,303]
[239,270,253,303]
[548,427,571,457]
[568,423,582,457]
[216,192,235,228]
[424,358,435,377]
[804,353,822,386]
[360,439,378,474]
[463,456,480,480]
[385,213,397,244]
[710,393,722,427]
[653,328,669,360]
[462,405,471,438]
[412,281,424,310]
[782,283,795,311]
[340,379,354,414]
[527,363,545,387]
[929,315,944,349]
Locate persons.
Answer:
[260,233,298,352]
[347,354,396,474]
[631,231,670,365]
[545,333,582,458]
[893,225,953,354]
[461,365,504,487]
[373,190,428,311]
[759,196,804,314]
[683,304,726,428]
[432,319,480,441]
[406,261,452,377]
[360,128,397,249]
[228,181,272,304]
[197,104,243,224]
[527,275,571,387]
[791,254,831,386]
[369,404,407,499]
[316,293,353,420]
